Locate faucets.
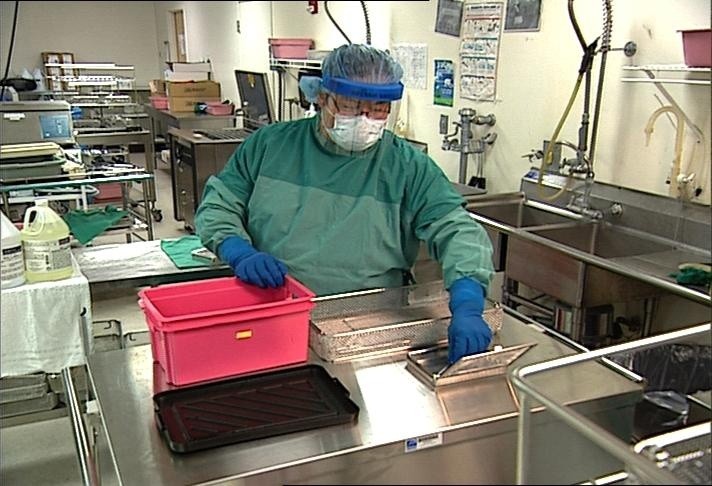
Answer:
[644,106,685,196]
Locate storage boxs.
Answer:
[680,28,709,69]
[268,37,311,59]
[138,275,316,390]
[148,80,221,113]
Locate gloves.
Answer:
[446,276,493,363]
[218,234,287,290]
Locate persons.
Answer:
[194,44,495,364]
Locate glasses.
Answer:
[331,94,392,121]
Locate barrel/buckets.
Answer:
[0,206,22,289]
[21,196,72,282]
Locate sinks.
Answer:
[524,222,677,259]
[466,197,586,230]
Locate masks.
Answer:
[320,106,388,152]
